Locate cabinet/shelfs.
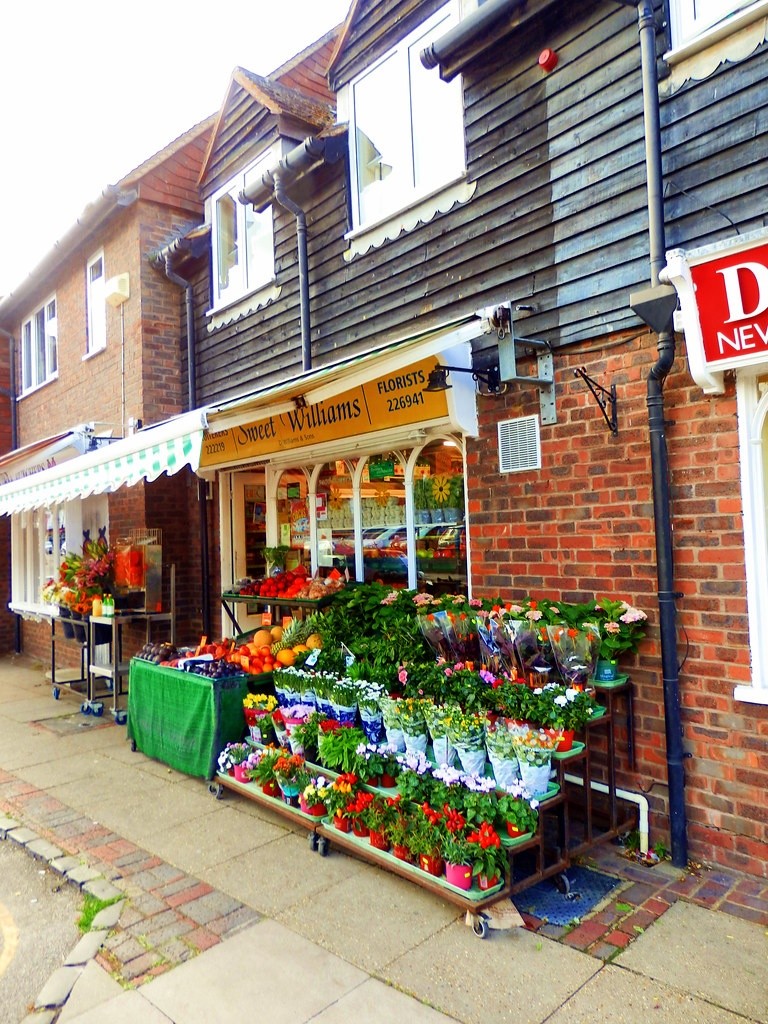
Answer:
[50,616,89,699]
[89,562,178,712]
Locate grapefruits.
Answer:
[276,634,321,666]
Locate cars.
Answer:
[328,521,467,570]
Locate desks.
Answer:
[126,657,250,782]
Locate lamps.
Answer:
[423,363,501,395]
[85,435,123,454]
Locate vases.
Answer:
[445,861,471,890]
[260,776,279,797]
[300,791,312,815]
[369,829,390,851]
[283,795,298,806]
[381,774,396,788]
[334,816,351,833]
[310,801,327,817]
[419,851,441,877]
[352,817,369,837]
[508,822,525,838]
[394,842,413,864]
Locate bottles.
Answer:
[92,593,115,617]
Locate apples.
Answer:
[240,572,296,596]
[205,643,283,675]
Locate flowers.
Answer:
[217,580,648,884]
[413,472,465,508]
[40,539,114,612]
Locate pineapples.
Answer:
[272,617,319,656]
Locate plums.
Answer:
[190,659,243,679]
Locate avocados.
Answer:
[135,643,184,664]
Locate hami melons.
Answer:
[247,620,292,647]
